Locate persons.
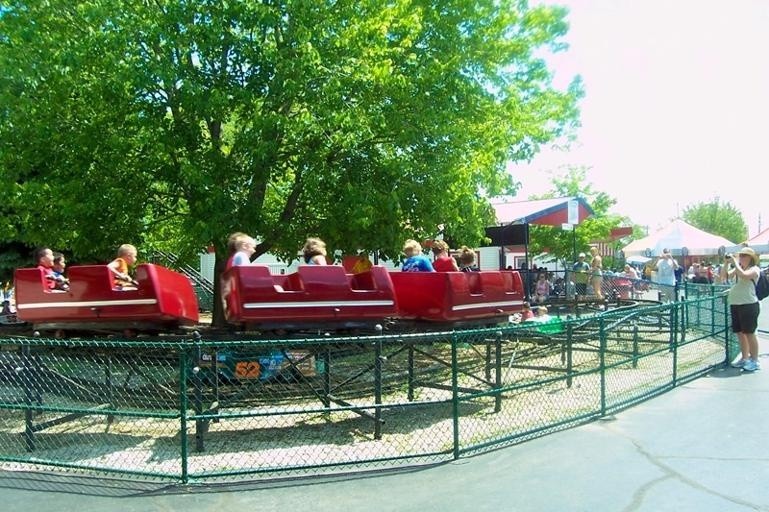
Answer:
[431,239,459,272]
[508,263,573,302]
[107,243,138,287]
[35,248,70,290]
[353,253,373,273]
[688,261,721,286]
[458,245,481,272]
[226,233,256,267]
[720,247,759,371]
[644,248,684,303]
[603,265,639,290]
[403,239,437,273]
[572,247,604,299]
[303,237,328,266]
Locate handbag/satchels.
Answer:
[751,265,769,300]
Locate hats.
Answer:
[736,247,759,265]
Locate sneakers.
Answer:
[731,359,761,371]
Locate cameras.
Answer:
[726,254,731,258]
